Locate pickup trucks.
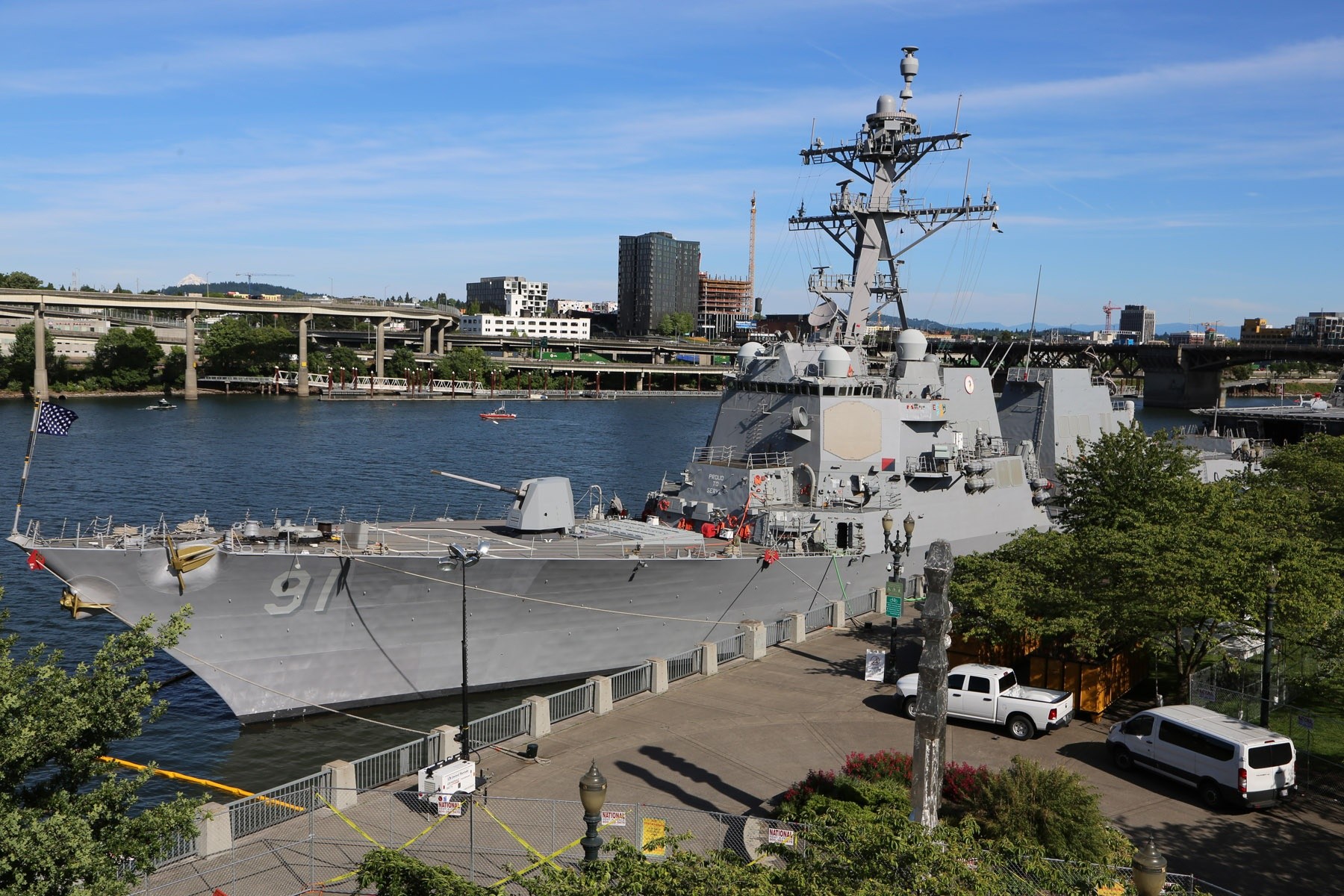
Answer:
[891,663,1076,741]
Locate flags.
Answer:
[36,400,79,436]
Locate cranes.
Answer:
[747,199,758,321]
[1102,300,1123,333]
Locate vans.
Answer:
[1105,703,1299,812]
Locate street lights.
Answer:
[578,756,608,874]
[880,509,915,681]
[436,539,494,760]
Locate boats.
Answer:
[1189,369,1344,442]
[145,405,178,410]
[479,413,518,420]
[8,44,1275,728]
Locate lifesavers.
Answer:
[803,484,811,497]
[660,500,668,511]
[728,516,737,528]
[1042,480,1050,489]
